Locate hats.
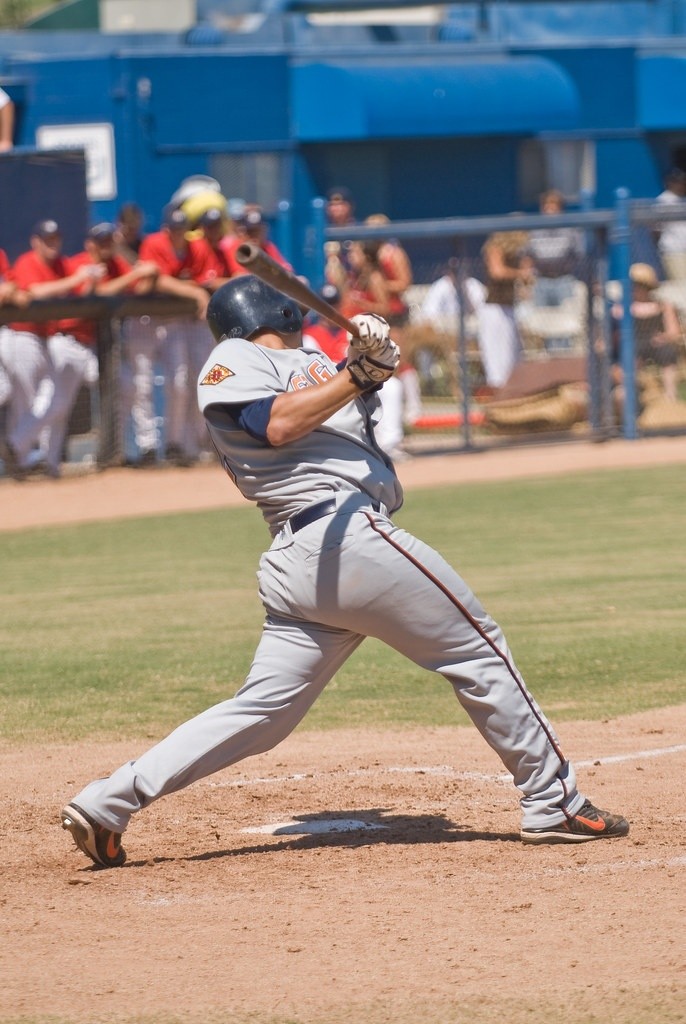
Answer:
[325,185,354,207]
[228,197,244,217]
[86,222,115,242]
[31,219,61,237]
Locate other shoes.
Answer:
[0,442,198,480]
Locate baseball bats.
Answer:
[235,242,360,336]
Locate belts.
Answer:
[289,498,379,534]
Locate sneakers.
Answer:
[60,802,127,867]
[521,798,630,843]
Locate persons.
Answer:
[0,172,685,476]
[57,277,629,865]
[0,88,13,156]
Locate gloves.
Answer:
[345,312,391,392]
[346,339,401,390]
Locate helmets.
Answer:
[206,274,310,345]
[233,205,273,229]
[627,262,660,289]
